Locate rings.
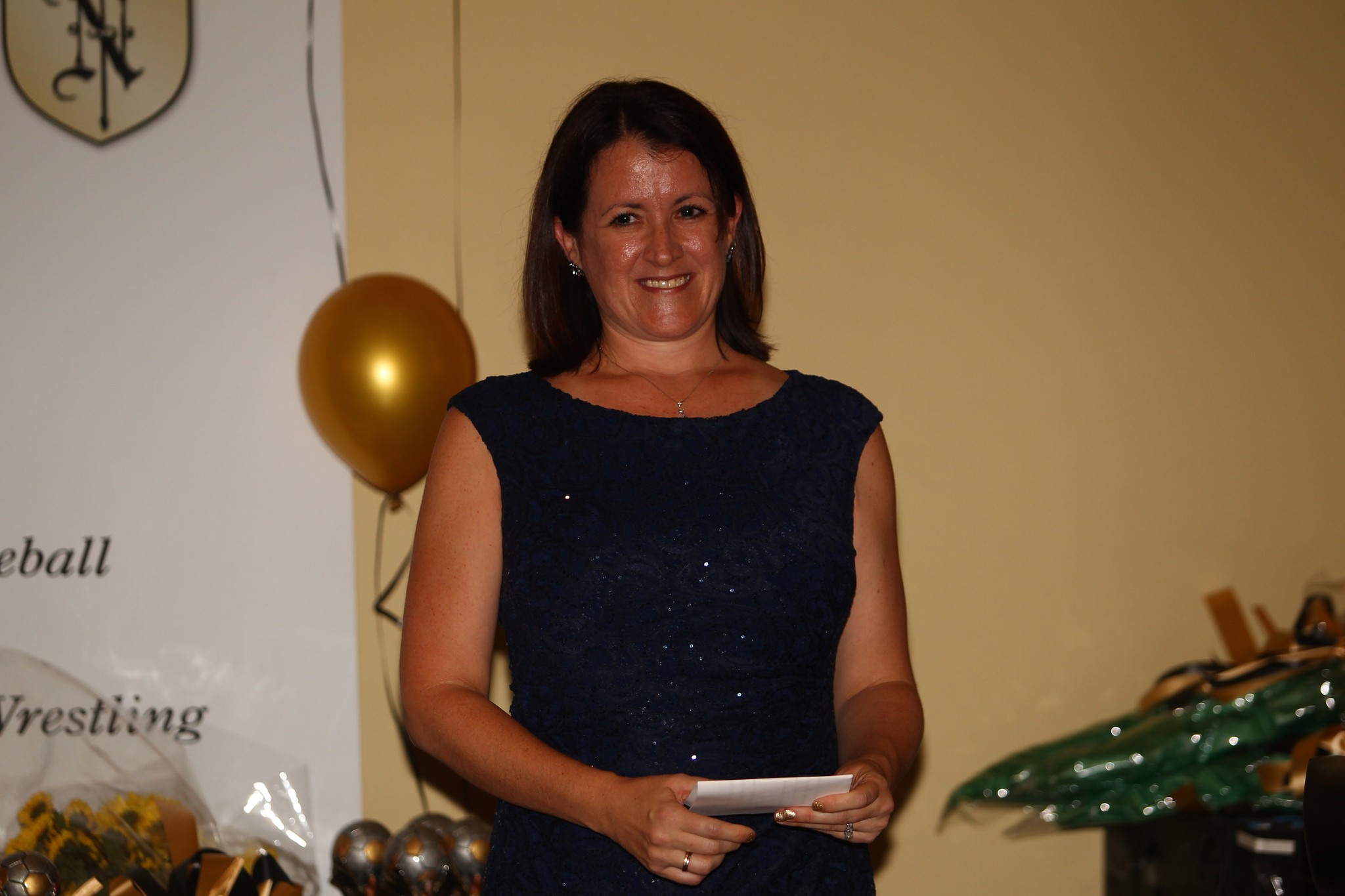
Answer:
[682,852,692,872]
[843,823,853,840]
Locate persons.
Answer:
[390,71,924,896]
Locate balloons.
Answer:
[293,269,479,510]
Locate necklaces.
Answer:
[594,338,733,416]
